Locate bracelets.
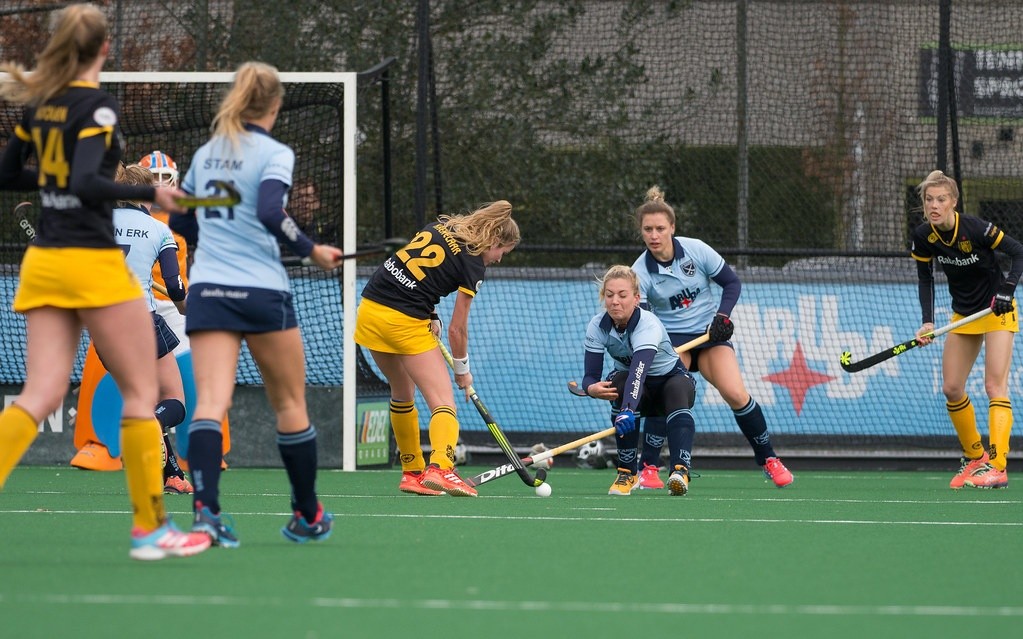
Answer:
[453,352,470,376]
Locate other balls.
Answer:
[536,484,552,497]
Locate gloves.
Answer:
[615,408,635,438]
[989,291,1015,315]
[705,312,738,343]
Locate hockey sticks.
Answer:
[434,336,547,484]
[472,426,618,486]
[176,180,244,210]
[838,306,994,373]
[331,237,406,259]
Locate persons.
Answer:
[630,185,794,488]
[70,149,231,496]
[168,61,344,549]
[911,170,1023,490]
[354,201,521,497]
[582,266,697,495]
[0,3,212,560]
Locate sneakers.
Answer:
[761,456,794,488]
[281,501,334,543]
[666,464,689,496]
[607,467,640,495]
[190,504,241,548]
[949,451,1008,490]
[638,462,664,488]
[129,520,211,561]
[399,464,479,498]
[162,473,193,495]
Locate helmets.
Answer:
[137,150,179,209]
